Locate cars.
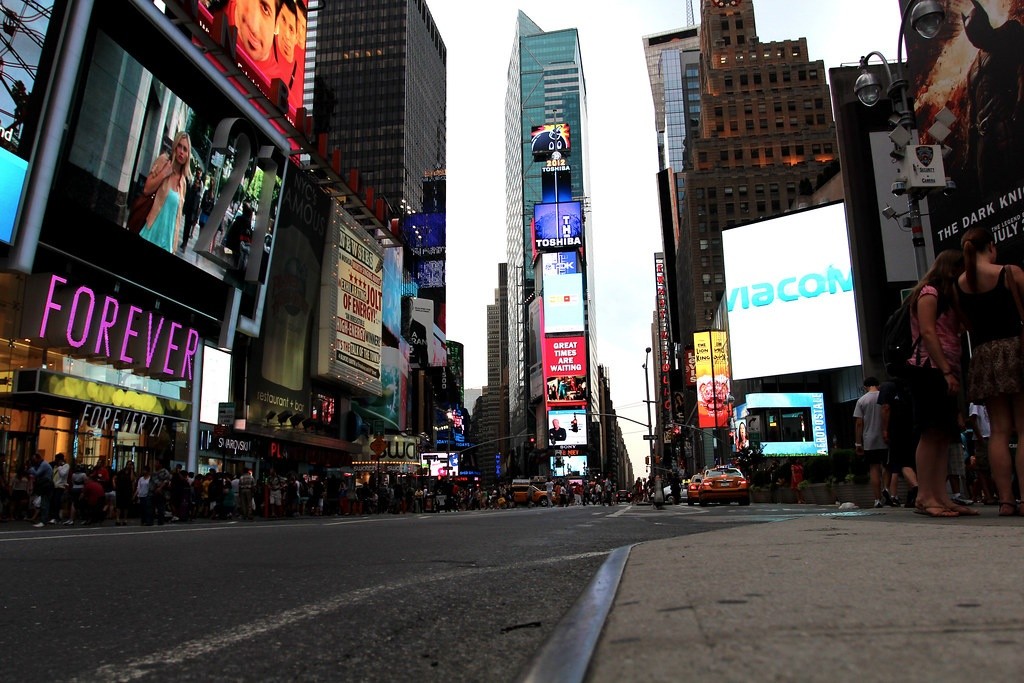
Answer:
[684,473,702,507]
[695,466,750,507]
[650,480,689,504]
[616,490,631,504]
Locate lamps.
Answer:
[265,410,337,436]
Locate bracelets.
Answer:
[943,371,952,376]
[855,443,861,446]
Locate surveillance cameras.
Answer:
[892,182,906,195]
[943,181,956,195]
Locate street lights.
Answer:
[641,347,655,481]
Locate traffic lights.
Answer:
[571,420,579,432]
[673,427,679,438]
[529,436,536,444]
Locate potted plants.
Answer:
[751,448,909,506]
[948,473,965,493]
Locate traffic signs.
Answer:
[443,467,453,470]
[643,434,658,440]
[439,458,449,462]
[423,455,439,460]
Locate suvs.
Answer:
[511,484,557,508]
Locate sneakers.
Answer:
[34,522,45,527]
[873,500,883,508]
[956,496,974,505]
[50,519,57,524]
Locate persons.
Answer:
[738,422,749,449]
[947,401,999,506]
[905,249,980,518]
[544,474,611,508]
[952,224,1024,517]
[11,453,514,528]
[180,168,216,252]
[790,457,806,503]
[767,460,784,504]
[550,419,566,441]
[139,131,194,256]
[549,376,586,400]
[853,377,901,508]
[670,473,683,505]
[226,0,307,109]
[527,482,534,502]
[877,380,918,507]
[961,0,1024,200]
[632,476,653,505]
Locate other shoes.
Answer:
[905,486,919,507]
[891,497,902,507]
[64,519,88,526]
[116,521,127,526]
[547,505,553,508]
[574,503,582,506]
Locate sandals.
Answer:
[998,502,1018,516]
[1018,502,1024,516]
[950,506,980,516]
[913,505,960,517]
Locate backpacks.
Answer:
[881,300,922,375]
